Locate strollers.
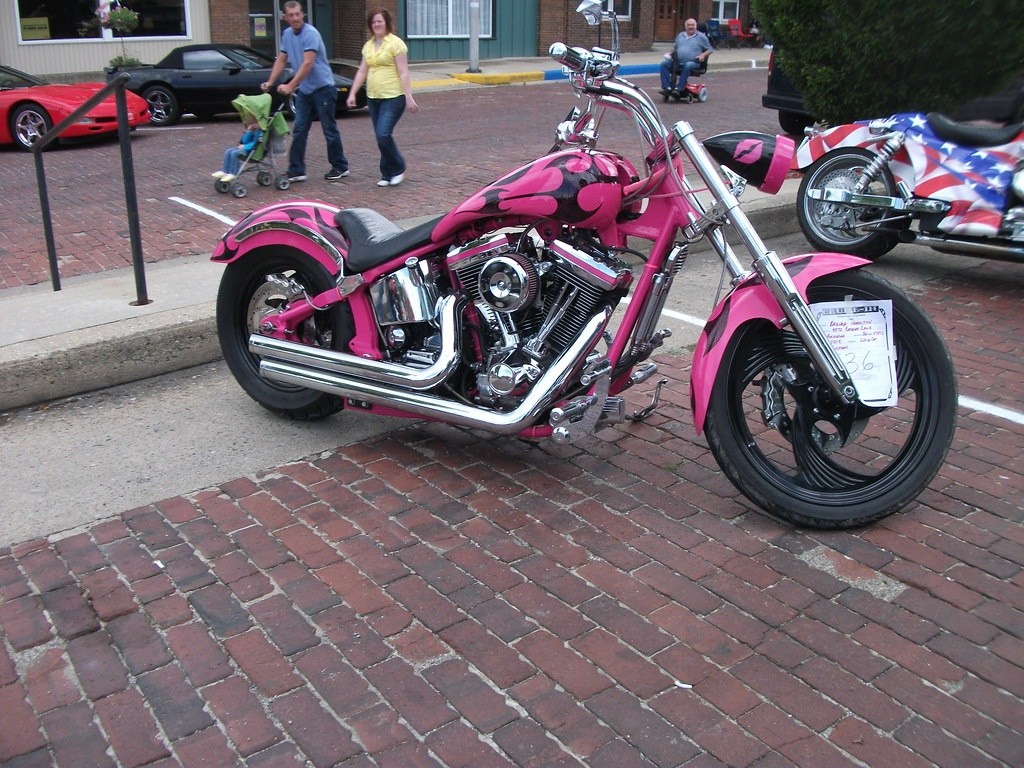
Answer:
[215,95,290,198]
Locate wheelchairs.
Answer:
[658,51,708,104]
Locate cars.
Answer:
[762,38,824,134]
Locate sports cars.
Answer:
[0,64,152,152]
[105,43,366,127]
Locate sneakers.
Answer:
[377,173,404,187]
[325,167,349,179]
[288,171,306,181]
[211,171,236,182]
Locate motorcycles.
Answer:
[210,1,957,530]
[787,111,1024,261]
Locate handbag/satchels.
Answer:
[269,131,287,158]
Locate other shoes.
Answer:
[764,45,773,49]
[658,89,680,99]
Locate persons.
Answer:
[750,23,760,49]
[211,113,263,182]
[658,19,713,100]
[260,0,349,182]
[346,6,421,186]
